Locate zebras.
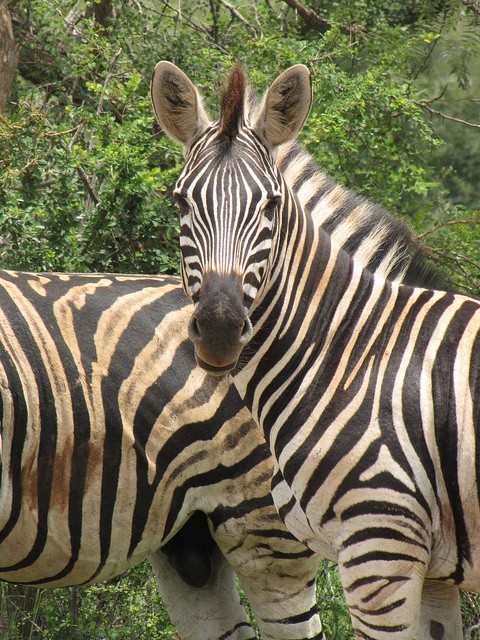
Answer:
[150,60,480,640]
[0,267,332,640]
